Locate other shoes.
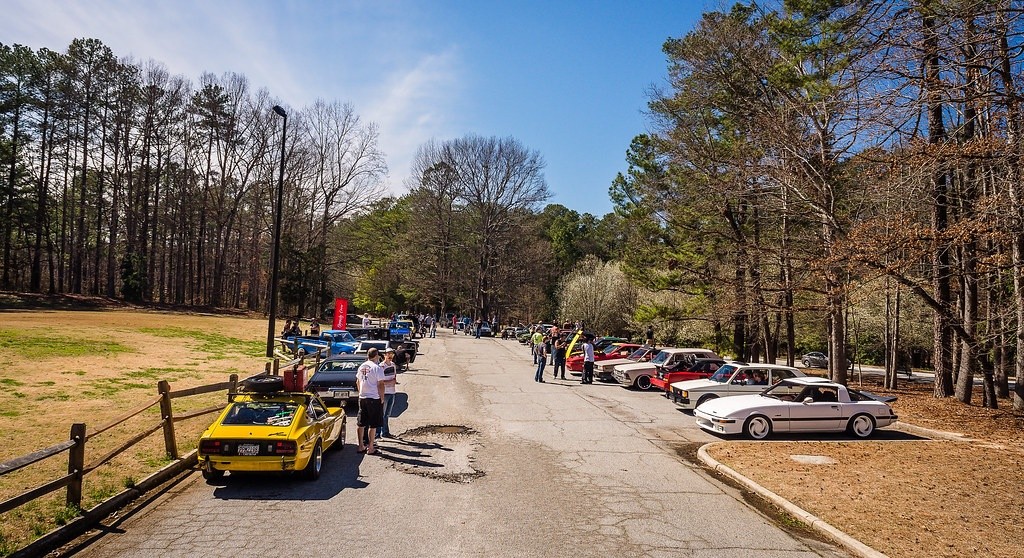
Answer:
[381,432,396,438]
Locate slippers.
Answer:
[358,448,369,453]
[367,450,382,455]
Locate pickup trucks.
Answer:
[286,330,360,360]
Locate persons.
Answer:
[534,337,547,383]
[355,348,385,454]
[413,311,437,338]
[362,313,372,328]
[549,324,560,365]
[645,325,654,346]
[374,347,397,438]
[552,333,570,380]
[580,338,594,384]
[501,328,509,340]
[474,315,482,339]
[563,319,585,330]
[492,315,498,337]
[282,319,301,352]
[463,316,470,335]
[529,321,546,364]
[310,319,320,336]
[392,311,404,328]
[452,314,458,335]
[743,370,755,385]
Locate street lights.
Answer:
[267,105,288,373]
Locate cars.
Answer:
[438,312,492,337]
[591,345,677,382]
[197,390,347,483]
[566,342,657,377]
[669,362,808,409]
[650,358,753,399]
[319,308,422,363]
[693,376,899,442]
[355,339,390,360]
[503,322,629,362]
[611,348,732,393]
[303,354,385,408]
[801,350,852,370]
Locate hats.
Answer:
[385,347,393,353]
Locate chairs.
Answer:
[238,407,258,423]
[823,390,835,401]
